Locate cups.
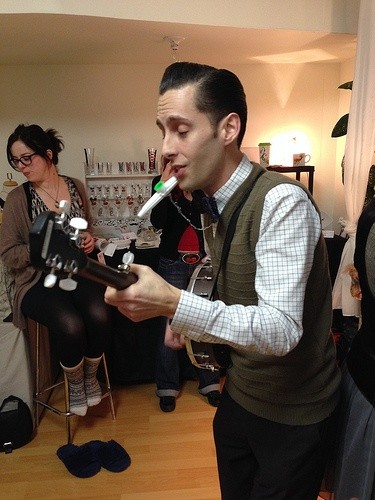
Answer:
[258,143,271,167]
[147,147,157,174]
[97,161,104,175]
[118,161,125,175]
[292,153,311,167]
[106,161,112,175]
[126,161,132,175]
[84,147,96,176]
[140,162,146,175]
[133,162,139,175]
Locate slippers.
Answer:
[56,443,101,479]
[84,441,131,472]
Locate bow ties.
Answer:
[196,196,218,222]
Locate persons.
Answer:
[0,124,102,417]
[148,156,220,414]
[99,62,343,500]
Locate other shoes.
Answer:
[160,395,175,412]
[209,389,220,407]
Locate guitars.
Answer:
[28,201,233,371]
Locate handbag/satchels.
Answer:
[0,395,32,454]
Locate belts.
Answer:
[175,252,202,264]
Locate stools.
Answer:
[34,322,116,444]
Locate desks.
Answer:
[88,234,349,386]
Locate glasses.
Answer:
[10,151,39,168]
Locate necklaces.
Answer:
[34,176,59,208]
